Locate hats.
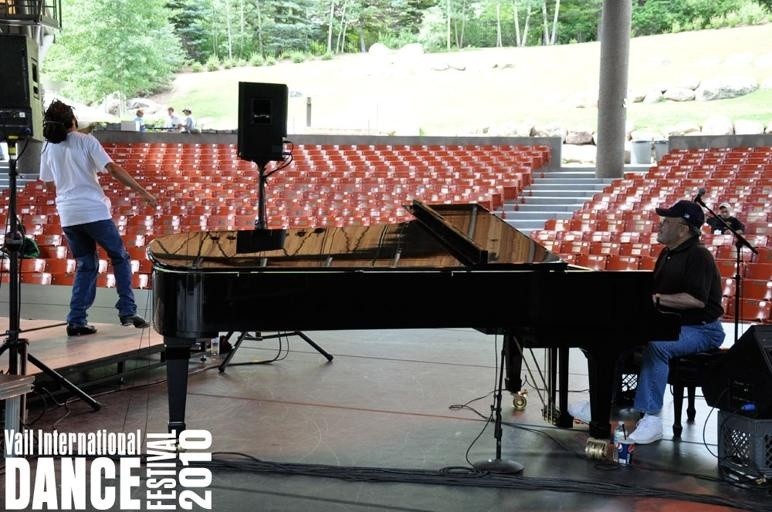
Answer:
[655,200,704,227]
[719,203,730,209]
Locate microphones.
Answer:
[693,188,706,203]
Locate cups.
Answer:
[617,439,635,467]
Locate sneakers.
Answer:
[568,399,591,424]
[628,414,663,444]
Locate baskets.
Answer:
[717,410,772,479]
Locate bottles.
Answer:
[613,421,628,463]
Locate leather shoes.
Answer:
[120,314,149,328]
[67,326,97,336]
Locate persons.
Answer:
[134,110,145,133]
[39,101,159,336]
[628,200,726,444]
[183,107,194,134]
[168,108,181,133]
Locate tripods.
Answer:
[0,159,103,413]
[219,168,334,373]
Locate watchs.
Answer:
[656,293,661,308]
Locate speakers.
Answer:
[702,325,772,419]
[237,81,288,164]
[0,34,45,143]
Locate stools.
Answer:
[639,344,716,445]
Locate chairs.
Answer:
[0,139,552,286]
[530,145,772,323]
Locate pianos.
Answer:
[143,199,684,463]
